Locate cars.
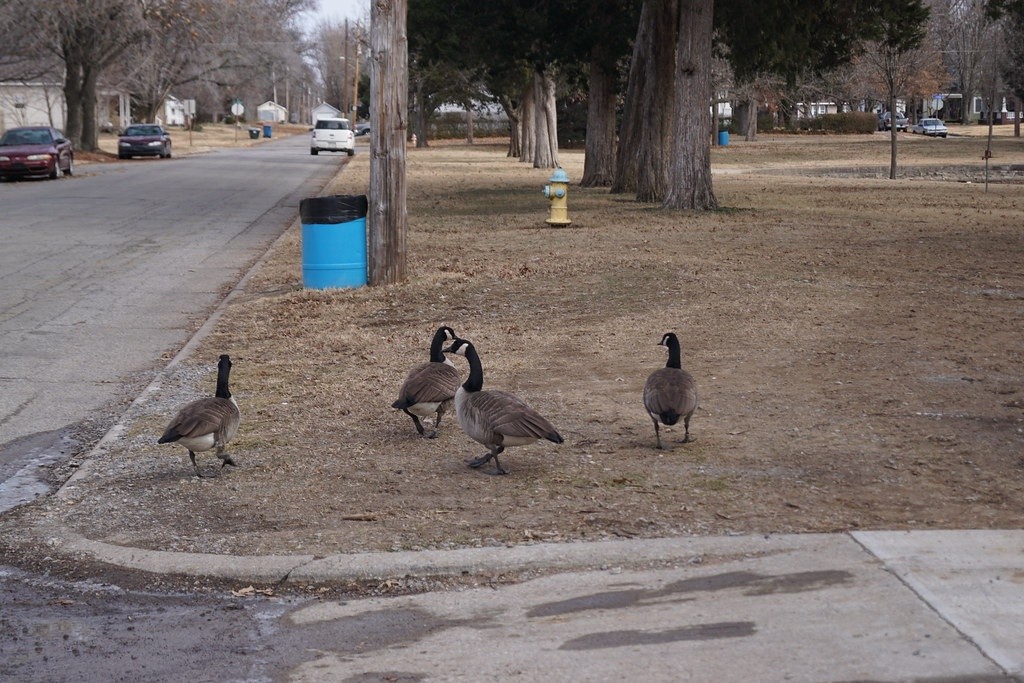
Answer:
[118,124,174,159]
[309,117,356,156]
[0,126,75,179]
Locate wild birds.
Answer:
[439,339,565,476]
[390,326,462,439]
[157,354,242,480]
[642,332,699,450]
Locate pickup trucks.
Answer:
[912,117,948,138]
[880,111,909,132]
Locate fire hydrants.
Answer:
[540,166,571,227]
[411,134,416,145]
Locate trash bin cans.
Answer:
[719,130,728,145]
[249,129,260,139]
[263,126,272,137]
[300,194,368,290]
